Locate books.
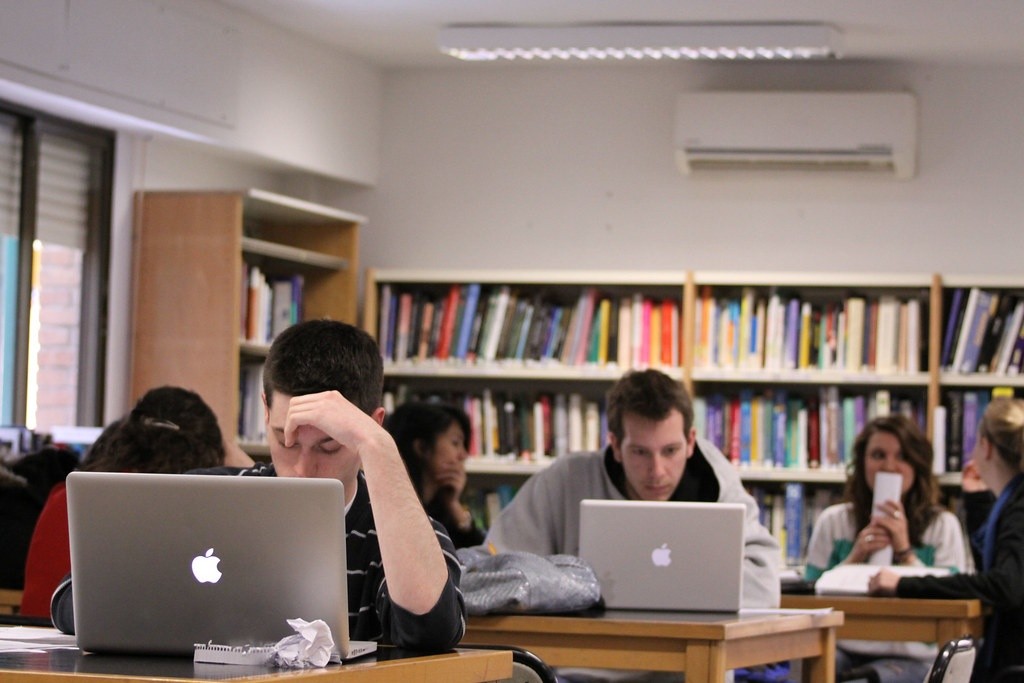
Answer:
[240,267,1024,565]
[0,427,103,460]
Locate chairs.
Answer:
[923,634,977,683]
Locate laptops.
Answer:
[578,498,746,619]
[65,470,377,659]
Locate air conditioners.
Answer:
[674,91,917,181]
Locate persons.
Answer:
[483,369,783,683]
[0,448,77,591]
[745,413,968,683]
[382,398,486,551]
[50,320,468,654]
[868,397,1024,683]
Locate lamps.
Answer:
[439,27,846,62]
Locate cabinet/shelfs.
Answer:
[128,190,368,454]
[363,267,1024,589]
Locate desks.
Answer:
[458,608,844,683]
[0,624,513,683]
[779,593,983,654]
